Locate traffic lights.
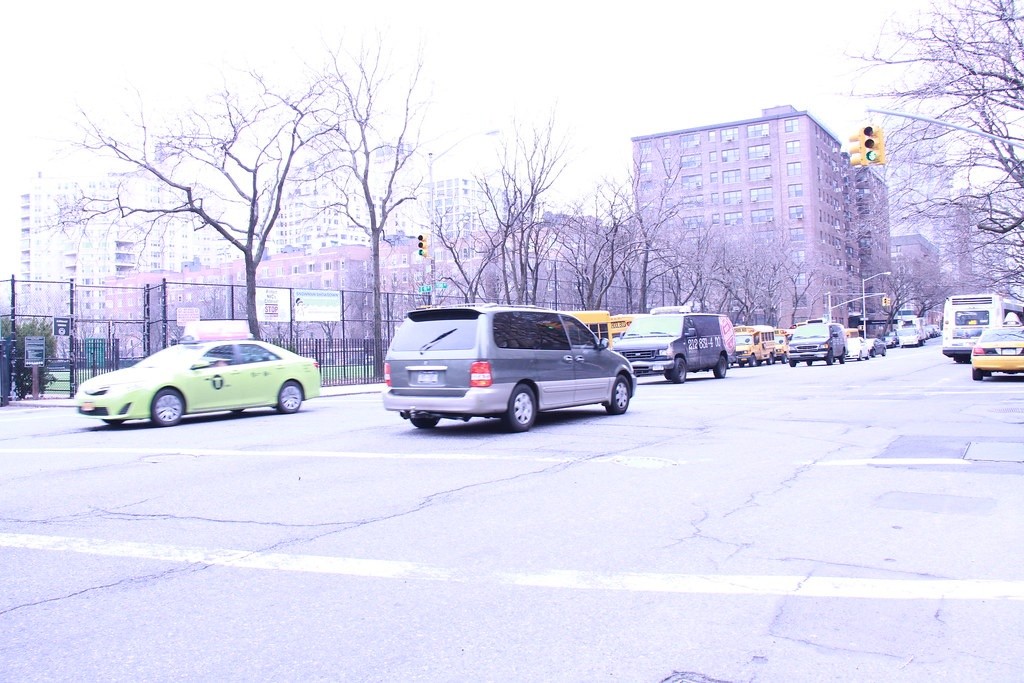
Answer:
[418,233,427,259]
[882,297,890,307]
[848,125,886,168]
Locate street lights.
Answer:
[429,128,503,304]
[862,272,891,338]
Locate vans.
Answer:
[614,314,735,384]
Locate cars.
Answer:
[843,337,886,361]
[882,326,939,348]
[971,325,1024,380]
[789,324,846,365]
[75,320,320,425]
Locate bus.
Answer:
[773,329,793,364]
[898,315,926,348]
[728,325,776,365]
[610,314,639,351]
[559,310,614,354]
[942,294,1024,362]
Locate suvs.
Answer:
[383,304,636,432]
